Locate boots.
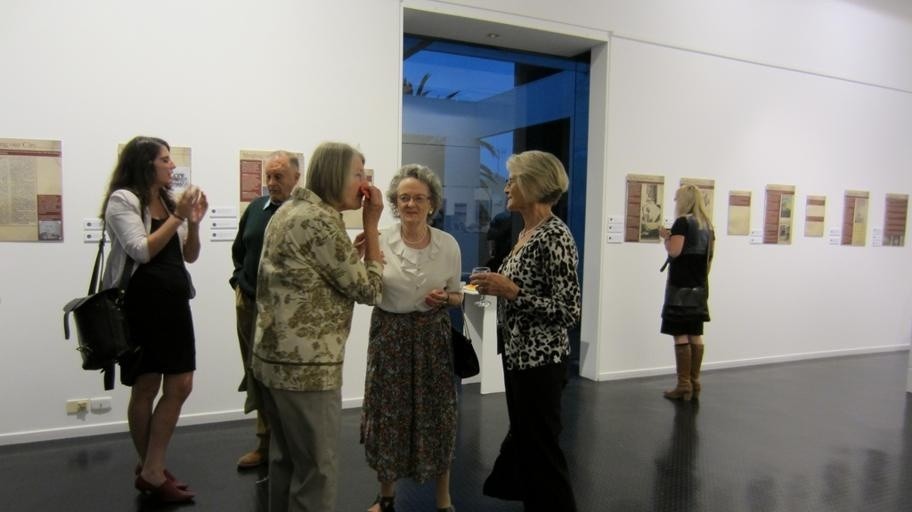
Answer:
[663,344,692,401]
[692,344,704,398]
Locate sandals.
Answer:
[366,491,395,512]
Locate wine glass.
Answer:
[471,267,492,309]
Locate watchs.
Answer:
[441,293,450,306]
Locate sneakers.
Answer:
[236,447,266,468]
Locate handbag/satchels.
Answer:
[670,286,704,316]
[452,332,480,378]
[63,287,152,370]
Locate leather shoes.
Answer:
[137,476,196,499]
[134,464,189,490]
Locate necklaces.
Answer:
[402,223,428,245]
[518,214,551,240]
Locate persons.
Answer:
[99,137,209,503]
[250,142,385,512]
[641,182,661,240]
[359,163,464,512]
[468,149,582,512]
[228,151,302,469]
[659,185,715,402]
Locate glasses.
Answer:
[398,193,431,201]
[505,176,517,186]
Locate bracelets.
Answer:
[171,212,185,222]
